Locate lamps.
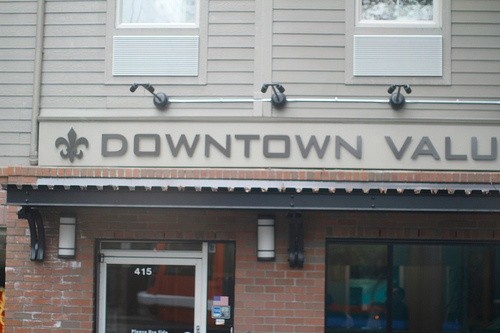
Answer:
[130,82,167,107]
[388,84,412,111]
[256,214,275,261]
[58,215,76,258]
[260,82,286,106]
